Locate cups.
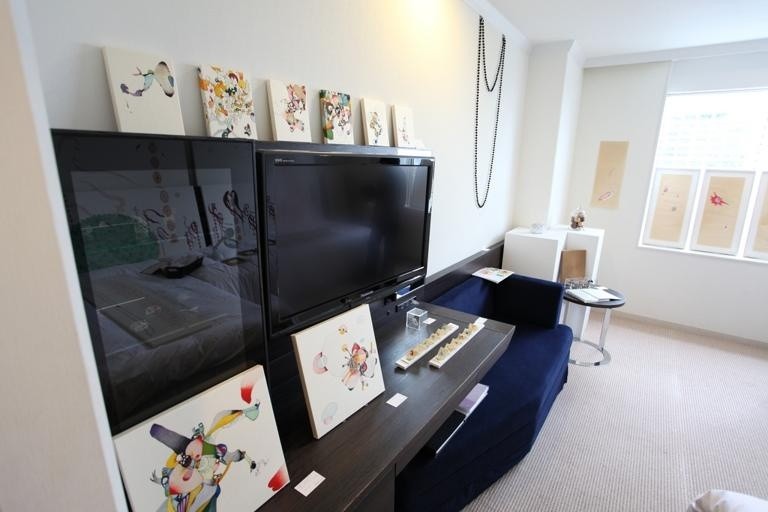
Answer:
[530,222,545,235]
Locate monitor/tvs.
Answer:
[257,148,435,340]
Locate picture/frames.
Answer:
[742,171,767,262]
[688,169,755,257]
[641,168,701,250]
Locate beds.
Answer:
[89,258,262,424]
[182,253,261,305]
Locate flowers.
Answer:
[570,210,586,230]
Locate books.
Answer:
[561,249,586,281]
[565,286,621,304]
[420,382,490,455]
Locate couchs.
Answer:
[398,273,573,512]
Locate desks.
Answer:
[563,281,626,366]
[256,297,516,512]
